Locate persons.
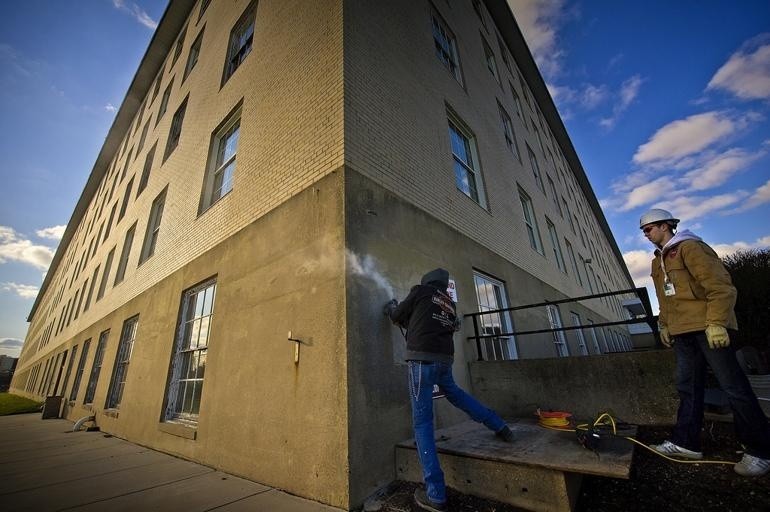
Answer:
[387,268,517,512]
[639,209,770,478]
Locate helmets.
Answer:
[639,209,680,228]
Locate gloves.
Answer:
[387,299,398,312]
[705,324,730,349]
[660,328,675,348]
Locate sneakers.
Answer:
[734,452,769,477]
[495,426,512,439]
[414,487,447,512]
[649,440,702,460]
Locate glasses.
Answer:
[643,224,657,233]
